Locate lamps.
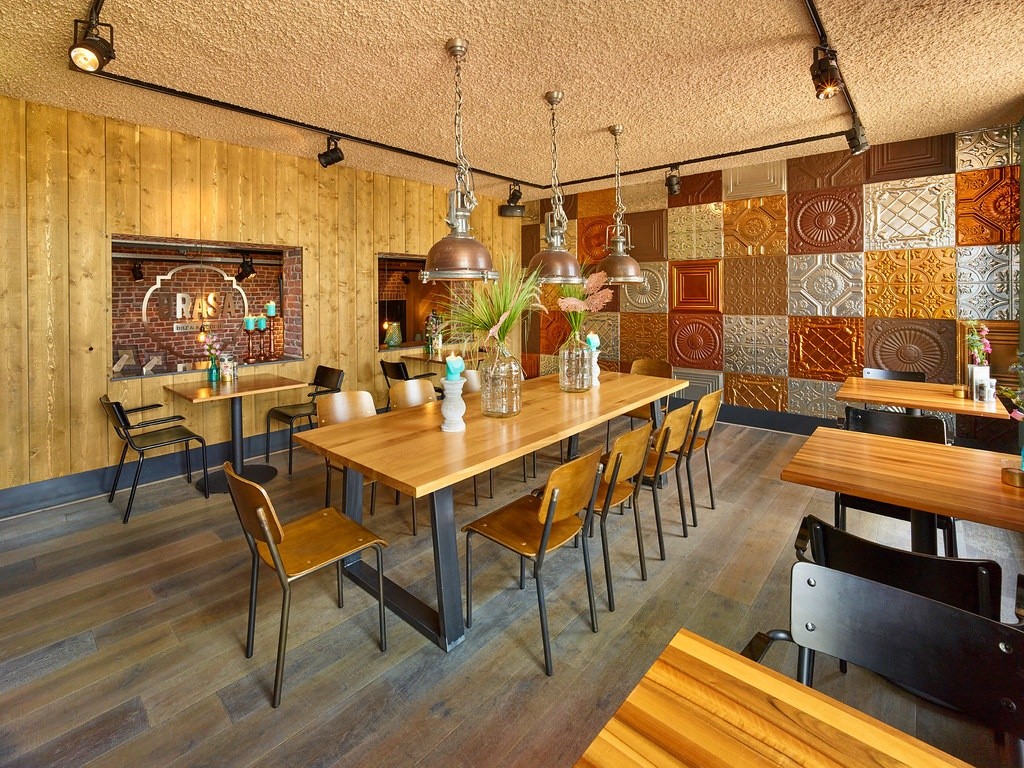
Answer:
[132,260,145,282]
[68,15,117,73]
[234,254,257,282]
[318,135,344,167]
[809,45,845,100]
[844,114,870,156]
[403,38,683,285]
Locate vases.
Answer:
[968,364,989,395]
[478,341,521,418]
[559,331,594,394]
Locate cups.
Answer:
[1001,458,1024,487]
[222,374,232,381]
[954,384,968,398]
[979,379,996,401]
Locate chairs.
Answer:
[100,347,1023,758]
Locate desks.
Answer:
[780,426,1024,555]
[573,628,980,768]
[291,368,689,653]
[835,377,1011,421]
[162,373,309,492]
[401,349,488,364]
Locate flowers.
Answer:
[426,320,440,336]
[989,350,1024,423]
[963,319,992,367]
[429,249,550,376]
[551,257,614,358]
[203,337,223,358]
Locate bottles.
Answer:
[232,362,238,379]
[207,355,221,382]
[424,333,433,354]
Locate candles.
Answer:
[586,333,601,352]
[243,301,275,330]
[445,352,464,381]
